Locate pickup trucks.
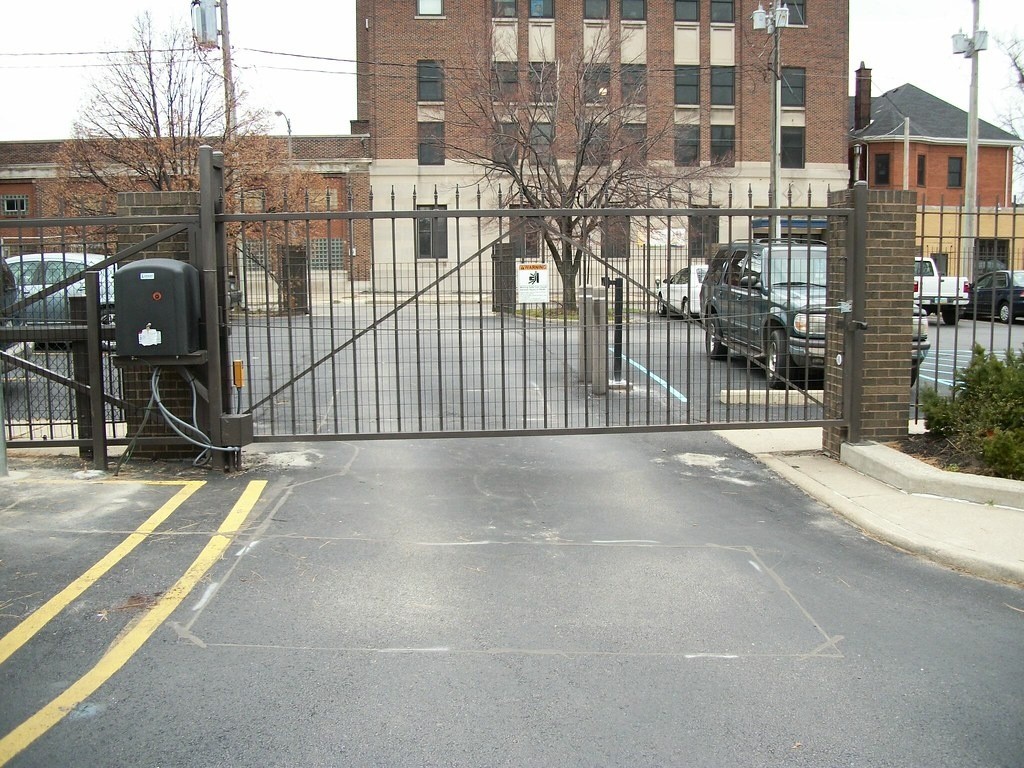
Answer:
[910,254,971,325]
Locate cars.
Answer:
[654,263,713,322]
[959,268,1023,325]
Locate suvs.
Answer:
[0,253,122,350]
[695,236,930,394]
